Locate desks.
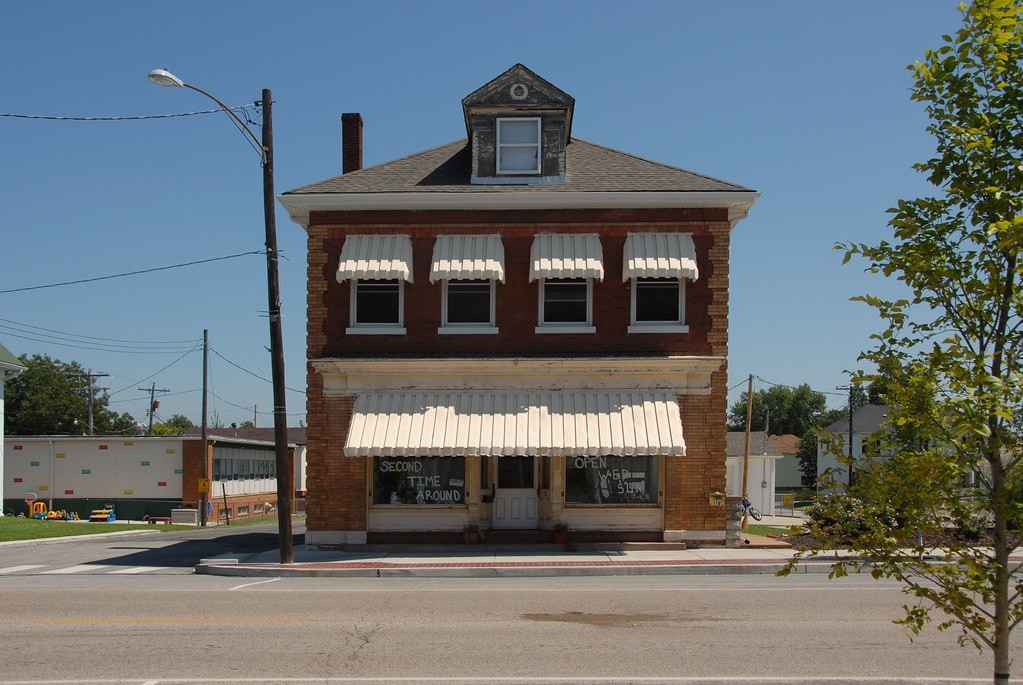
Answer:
[92,509,115,522]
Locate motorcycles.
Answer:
[741,493,763,521]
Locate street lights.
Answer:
[73,418,93,435]
[812,411,853,487]
[147,68,294,563]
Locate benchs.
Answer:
[89,515,116,521]
[147,517,173,524]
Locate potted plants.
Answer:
[462,520,479,544]
[552,522,567,544]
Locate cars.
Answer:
[32,502,47,520]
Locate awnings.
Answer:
[335,233,415,283]
[341,392,687,459]
[528,232,605,283]
[621,231,699,283]
[429,233,507,285]
